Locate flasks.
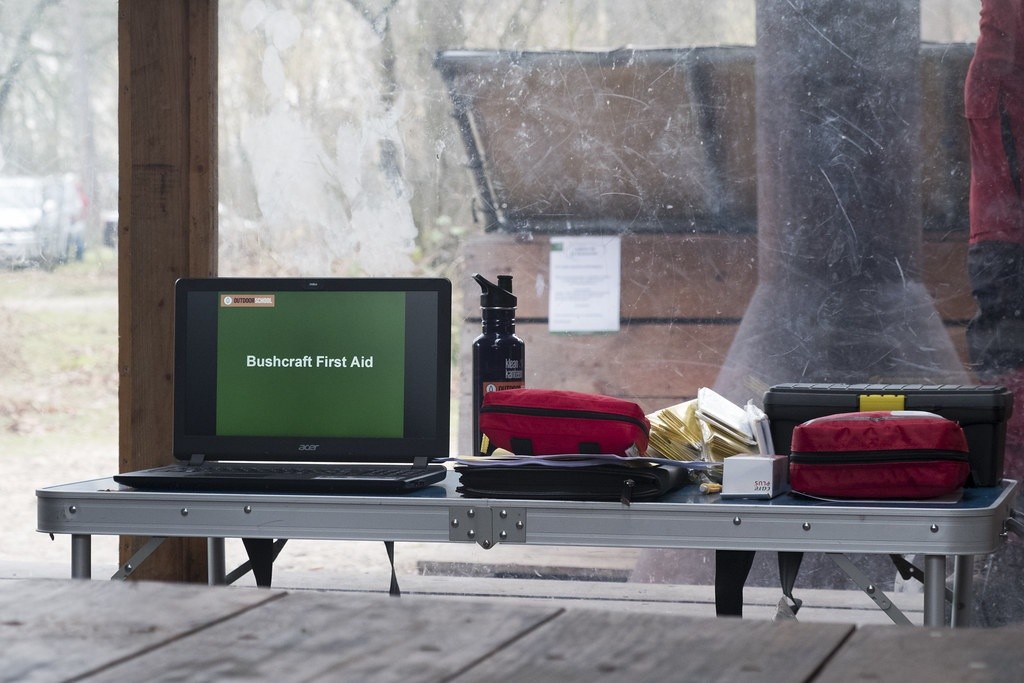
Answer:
[468,268,525,456]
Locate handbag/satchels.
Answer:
[453,457,689,503]
[787,410,970,501]
[479,388,651,458]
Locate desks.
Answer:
[37,461,1020,630]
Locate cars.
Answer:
[0,174,88,269]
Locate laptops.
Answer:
[113,275,451,493]
